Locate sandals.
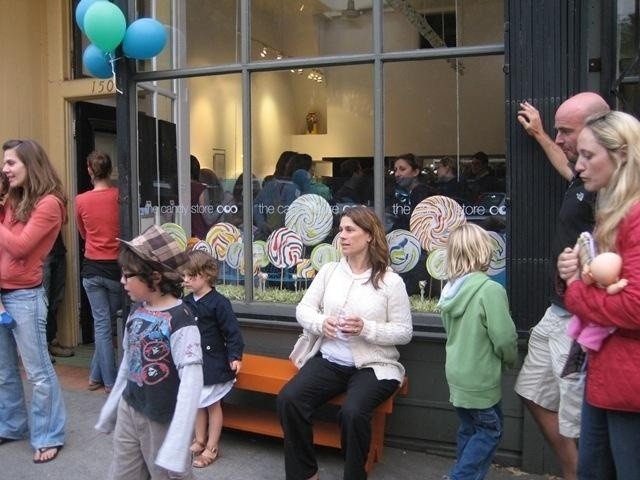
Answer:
[88,382,102,391]
[193,445,221,468]
[190,439,204,455]
[106,387,111,392]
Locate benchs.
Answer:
[223,354,408,476]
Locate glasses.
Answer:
[119,267,152,281]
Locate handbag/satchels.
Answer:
[289,260,341,372]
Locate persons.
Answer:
[71,150,124,395]
[431,222,520,480]
[191,154,335,241]
[556,109,640,480]
[335,160,364,205]
[569,232,629,356]
[434,156,466,200]
[511,91,611,480]
[385,153,432,234]
[0,139,68,465]
[180,250,246,467]
[514,242,587,480]
[471,151,506,194]
[274,205,413,480]
[93,222,205,480]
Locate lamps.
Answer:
[307,68,325,84]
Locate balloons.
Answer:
[83,1,126,54]
[74,0,91,36]
[122,17,168,62]
[82,44,116,81]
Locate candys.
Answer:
[192,240,212,255]
[160,223,187,252]
[409,196,466,295]
[293,274,298,292]
[206,222,242,286]
[310,243,340,272]
[386,229,422,274]
[253,240,270,292]
[258,272,262,290]
[226,242,244,288]
[485,230,506,288]
[262,273,268,291]
[185,238,201,254]
[331,231,348,261]
[266,228,303,290]
[427,248,450,298]
[285,193,333,260]
[297,259,316,290]
[418,281,428,302]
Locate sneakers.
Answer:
[0,312,14,328]
[49,341,74,357]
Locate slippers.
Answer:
[34,446,59,463]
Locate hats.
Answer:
[116,224,189,283]
